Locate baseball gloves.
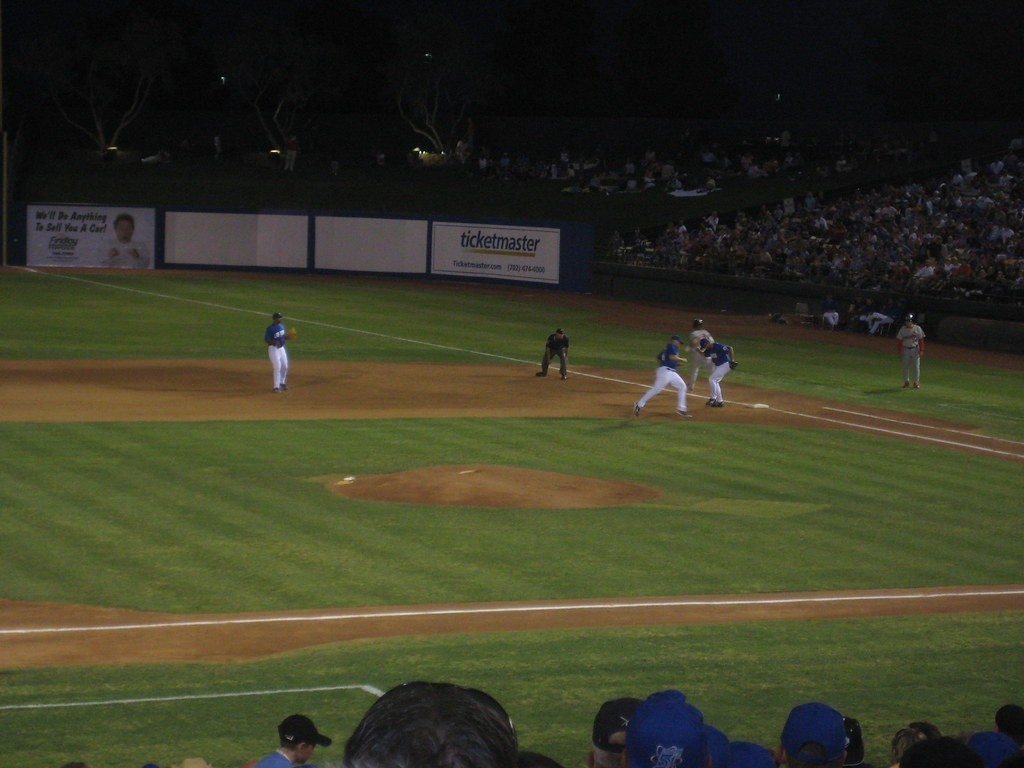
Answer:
[729,361,739,369]
[287,326,297,340]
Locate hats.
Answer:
[780,702,849,763]
[278,715,332,747]
[272,312,284,319]
[592,689,775,768]
[842,717,864,767]
[672,336,684,344]
[700,339,708,350]
[968,732,1020,768]
[557,328,565,334]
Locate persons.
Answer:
[895,314,926,389]
[819,307,893,337]
[214,132,222,159]
[264,313,296,393]
[633,335,693,419]
[180,681,1024,768]
[695,338,738,407]
[378,148,385,168]
[448,126,1024,305]
[689,318,715,391]
[283,135,301,172]
[535,328,570,380]
[95,211,150,267]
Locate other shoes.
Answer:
[274,387,282,394]
[280,384,288,390]
[676,410,692,418]
[633,402,640,416]
[904,381,909,389]
[913,383,920,389]
[560,375,567,380]
[711,401,723,407]
[706,398,716,406]
[536,372,546,377]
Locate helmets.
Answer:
[904,313,914,321]
[694,319,703,328]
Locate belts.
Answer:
[905,346,918,349]
